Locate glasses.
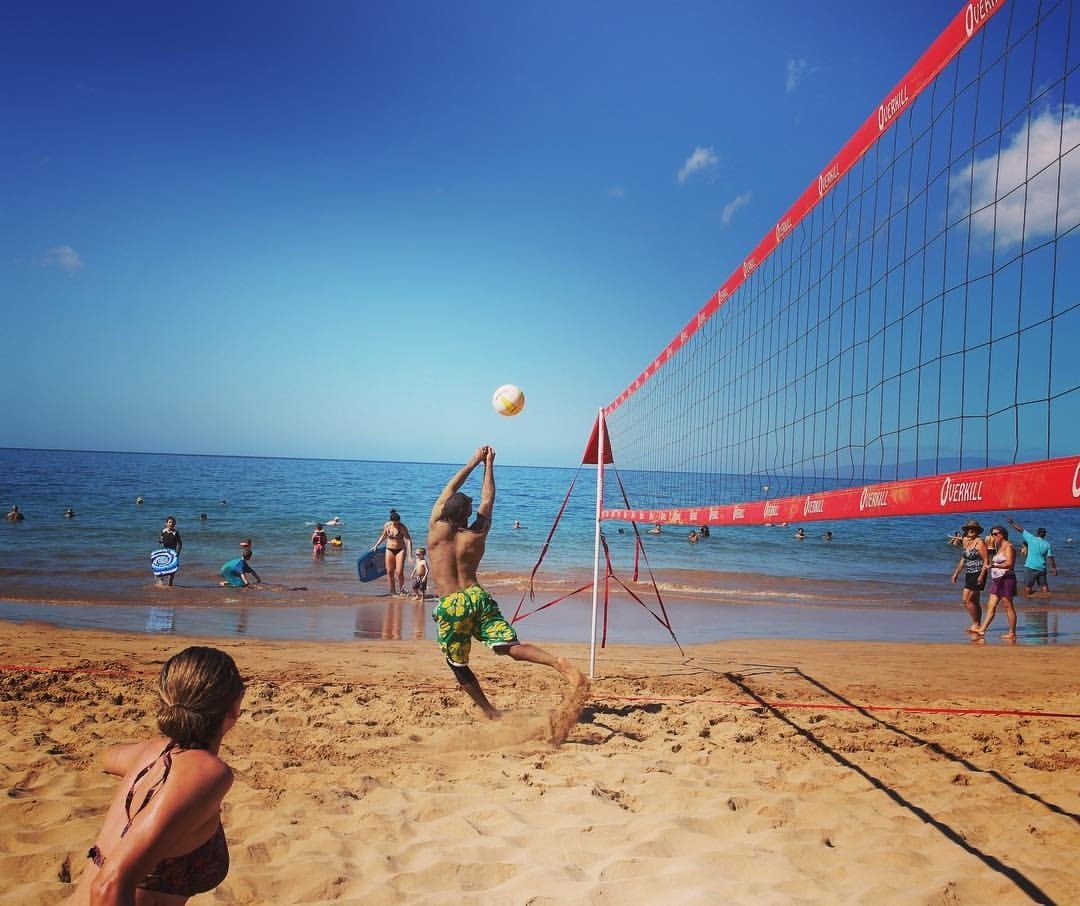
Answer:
[991,532,999,536]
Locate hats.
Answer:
[961,520,984,534]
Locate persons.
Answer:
[218,549,260,587]
[312,518,343,555]
[514,522,519,528]
[66,509,74,517]
[159,516,182,586]
[199,514,207,521]
[7,505,24,521]
[136,497,144,505]
[946,517,1058,639]
[426,446,572,721]
[616,521,833,544]
[78,646,244,906]
[370,510,430,602]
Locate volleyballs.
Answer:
[491,385,526,416]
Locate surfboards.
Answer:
[357,545,388,582]
